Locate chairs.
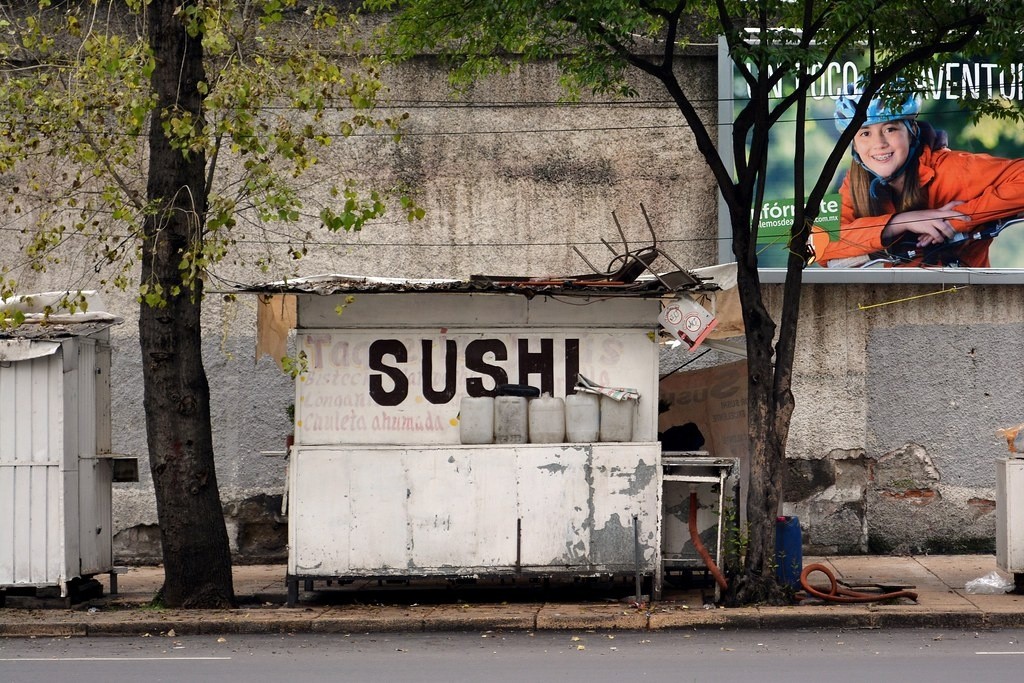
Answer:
[573,203,697,292]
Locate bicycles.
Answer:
[826,212,1024,268]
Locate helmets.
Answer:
[834,74,921,148]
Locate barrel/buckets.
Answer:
[774,515,802,592]
[461,390,634,445]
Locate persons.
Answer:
[805,73,1024,268]
